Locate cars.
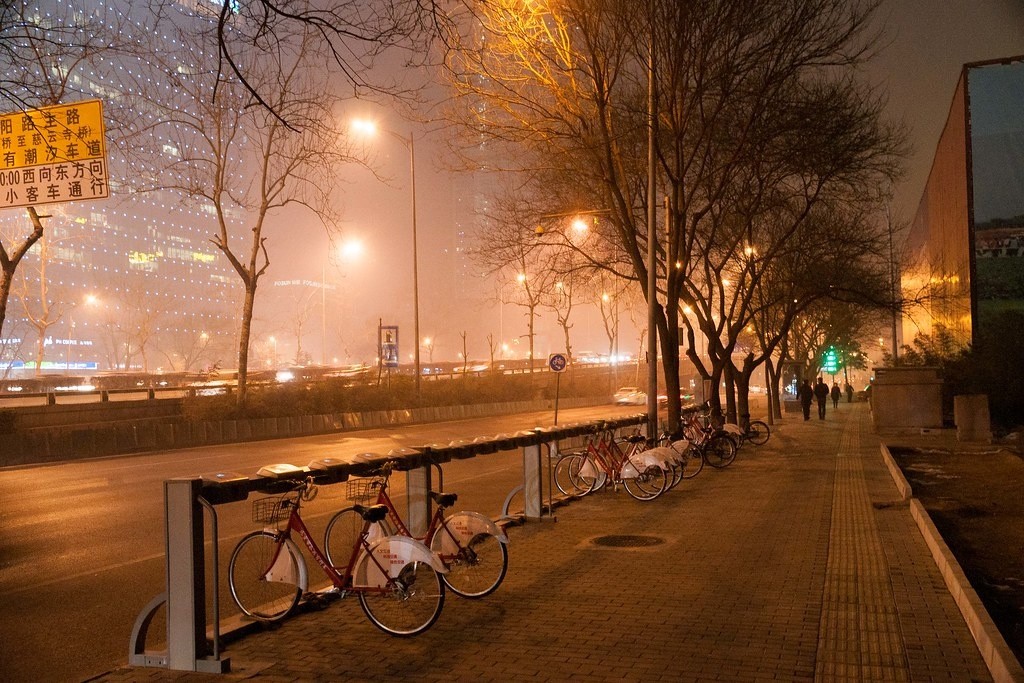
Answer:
[657,391,683,409]
[453,363,504,374]
[577,351,599,364]
[857,385,871,401]
[680,387,695,404]
[750,384,773,395]
[614,388,649,407]
[599,353,634,364]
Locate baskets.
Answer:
[251,496,293,523]
[346,476,384,502]
[565,418,681,454]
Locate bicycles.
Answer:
[554,406,771,501]
[226,473,452,636]
[324,460,509,603]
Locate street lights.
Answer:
[351,117,421,399]
[66,295,95,377]
[500,274,525,358]
[573,213,619,357]
[24,352,32,379]
[701,316,718,363]
[323,243,362,367]
[589,294,608,356]
[269,337,276,367]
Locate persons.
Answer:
[796,380,813,421]
[846,383,854,403]
[831,383,842,408]
[813,378,829,420]
